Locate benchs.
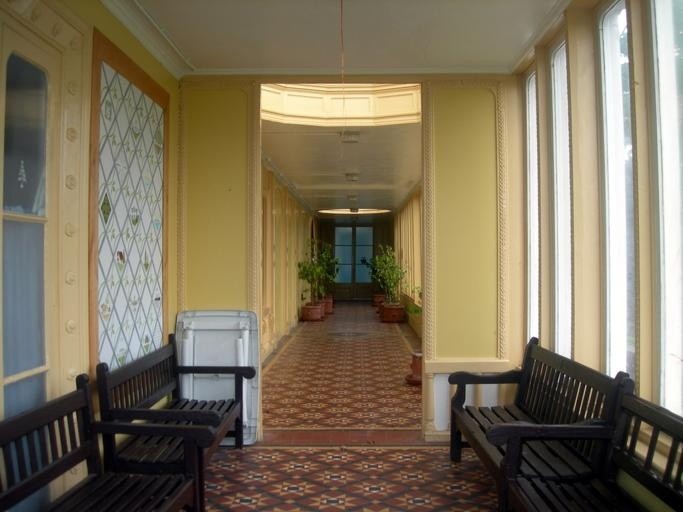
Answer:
[0,373,215,511]
[96,334,255,512]
[446,337,630,511]
[484,377,683,511]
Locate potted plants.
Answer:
[358,243,410,323]
[298,240,341,319]
